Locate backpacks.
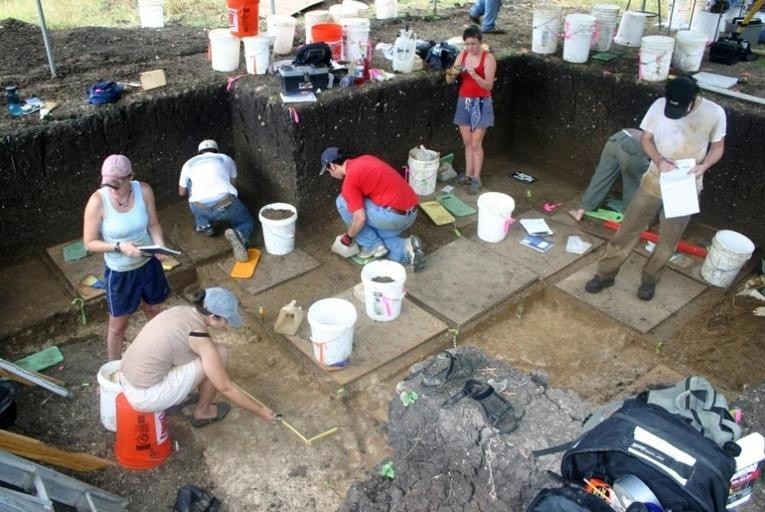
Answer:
[292,42,333,68]
[425,41,459,70]
[526,398,741,512]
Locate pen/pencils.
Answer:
[662,156,680,170]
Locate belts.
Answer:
[383,205,416,215]
[193,197,232,211]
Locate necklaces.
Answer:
[115,183,132,208]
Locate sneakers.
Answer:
[453,175,473,186]
[639,283,654,300]
[468,180,482,194]
[192,219,216,236]
[586,276,614,293]
[224,228,249,262]
[406,234,426,273]
[357,245,389,259]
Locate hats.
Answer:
[665,75,697,119]
[99,154,133,189]
[198,139,219,150]
[319,146,345,176]
[203,287,243,328]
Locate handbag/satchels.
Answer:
[646,375,741,449]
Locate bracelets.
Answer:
[115,241,121,253]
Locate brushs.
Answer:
[79,274,106,289]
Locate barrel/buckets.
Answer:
[97,359,122,432]
[137,0,165,29]
[407,145,440,196]
[476,191,516,243]
[306,297,359,368]
[700,229,756,289]
[360,259,407,322]
[258,202,298,256]
[114,392,180,469]
[208,0,765,82]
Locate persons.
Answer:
[178,139,254,262]
[569,128,651,222]
[469,0,504,34]
[118,284,281,428]
[585,75,727,300]
[446,25,497,194]
[83,154,171,363]
[319,147,426,273]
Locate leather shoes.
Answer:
[192,402,230,428]
[489,26,504,32]
[468,13,481,24]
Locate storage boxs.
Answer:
[278,61,330,97]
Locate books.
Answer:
[135,245,182,257]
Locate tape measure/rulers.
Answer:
[229,381,338,446]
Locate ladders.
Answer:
[0,448,132,512]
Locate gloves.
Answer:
[341,232,352,246]
